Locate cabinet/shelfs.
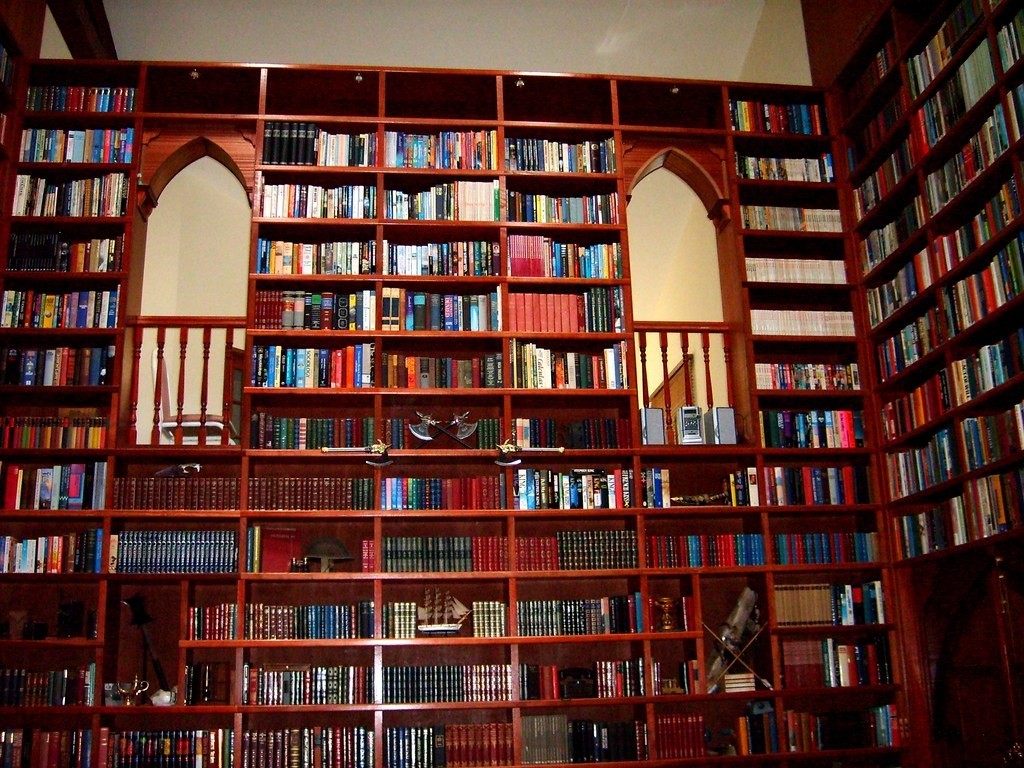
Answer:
[0,0,1024,768]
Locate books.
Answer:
[0,80,900,768]
[842,0,1024,556]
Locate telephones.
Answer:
[559,667,596,698]
